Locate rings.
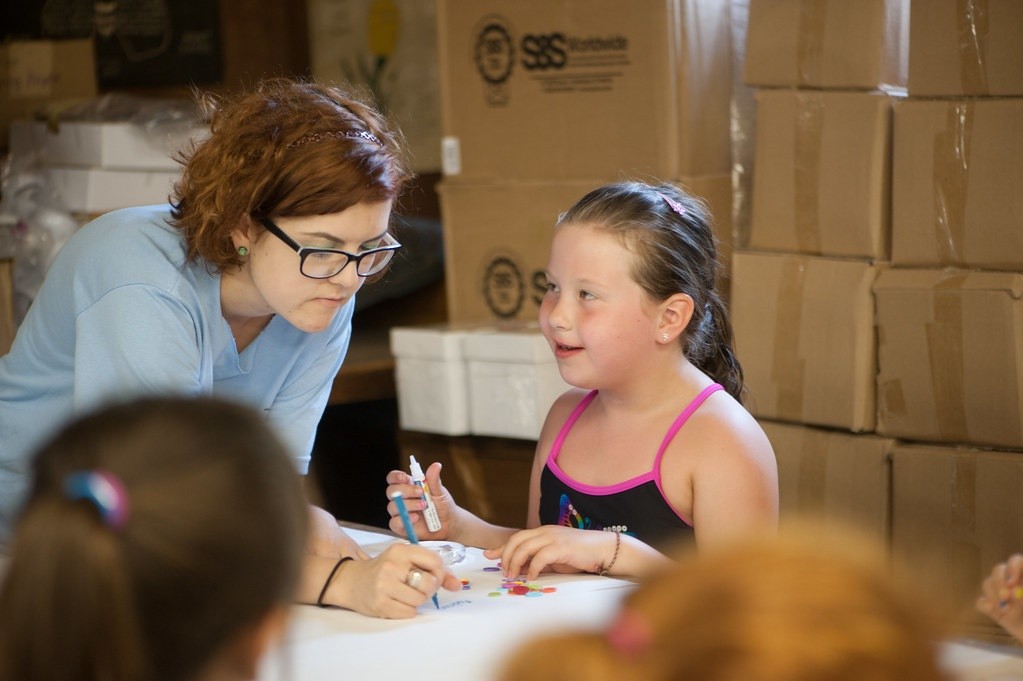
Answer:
[408,569,421,586]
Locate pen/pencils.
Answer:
[392,491,440,610]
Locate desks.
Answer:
[254,516,1023,681]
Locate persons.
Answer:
[0,80,466,680]
[975,552,1023,645]
[387,181,779,581]
[490,514,942,681]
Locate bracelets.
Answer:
[594,531,620,576]
[317,557,355,608]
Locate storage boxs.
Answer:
[0,0,1023,658]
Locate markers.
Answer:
[409,455,441,532]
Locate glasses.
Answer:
[260,218,402,279]
